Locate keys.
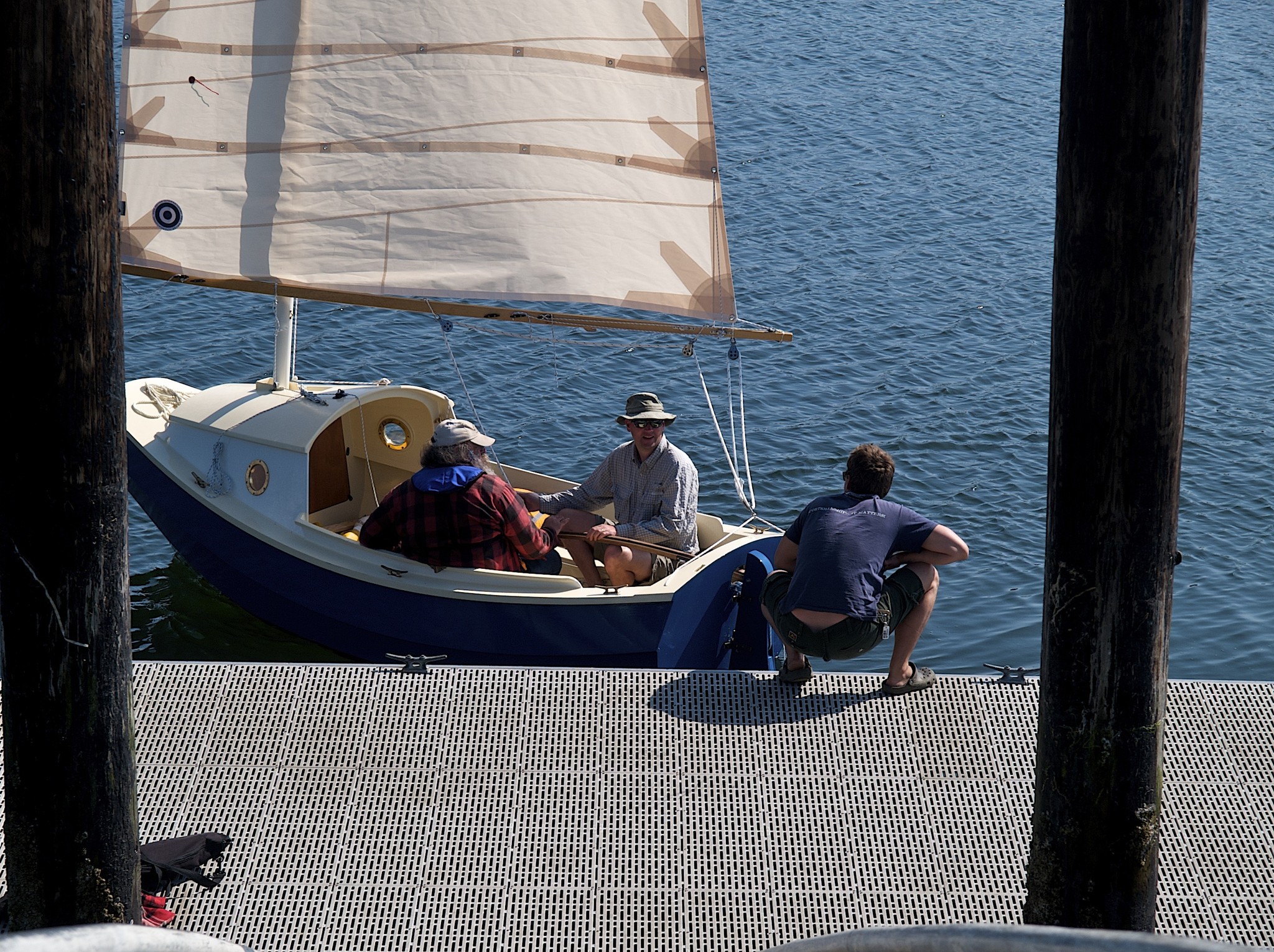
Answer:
[876,608,891,640]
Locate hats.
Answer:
[615,392,676,426]
[430,418,496,447]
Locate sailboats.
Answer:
[125,0,788,669]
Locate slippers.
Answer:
[778,654,813,680]
[881,660,936,694]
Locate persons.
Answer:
[759,444,969,694]
[359,418,570,576]
[515,393,701,588]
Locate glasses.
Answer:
[629,419,665,428]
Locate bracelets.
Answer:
[901,552,906,564]
